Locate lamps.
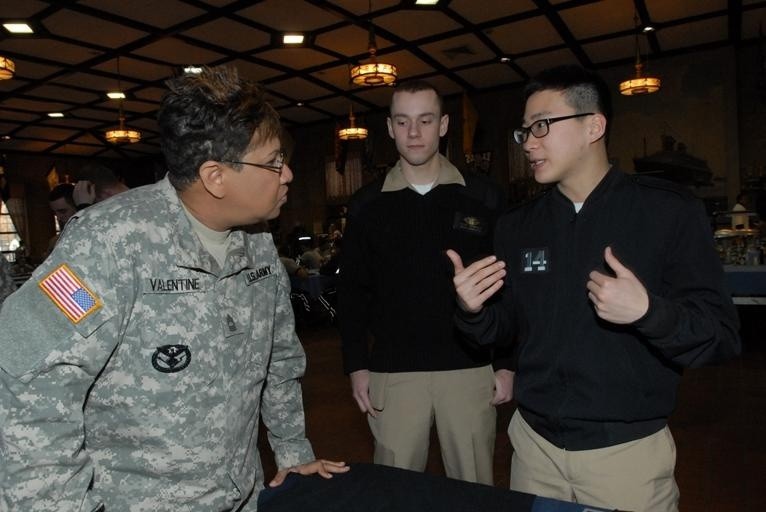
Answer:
[336,95,368,140]
[104,57,142,145]
[620,7,661,98]
[350,3,399,88]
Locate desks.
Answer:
[260,462,624,512]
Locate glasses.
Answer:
[512,111,595,144]
[216,150,288,170]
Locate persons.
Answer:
[446,63,742,512]
[339,81,517,486]
[274,240,328,276]
[0,65,351,512]
[48,170,129,225]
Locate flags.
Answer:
[42,267,97,320]
